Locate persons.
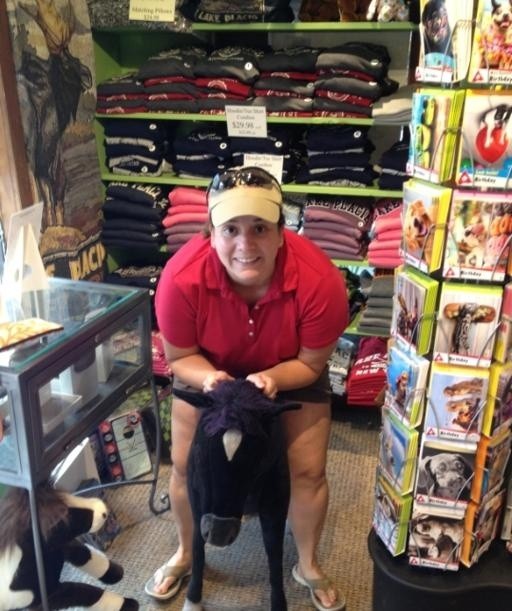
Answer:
[144,166,349,611]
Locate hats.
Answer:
[208,166,283,224]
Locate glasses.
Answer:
[213,170,268,190]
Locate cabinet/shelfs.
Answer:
[85,22,419,406]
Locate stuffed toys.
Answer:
[0,477,139,611]
[172,380,302,611]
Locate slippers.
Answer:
[144,558,193,599]
[291,564,347,611]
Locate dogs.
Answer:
[418,450,473,502]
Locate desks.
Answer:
[2,280,172,611]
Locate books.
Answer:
[370,0,512,564]
